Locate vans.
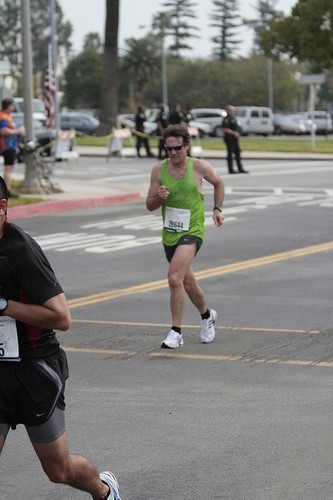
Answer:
[232,107,275,136]
[8,95,52,130]
[189,107,226,137]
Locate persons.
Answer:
[145,125,225,350]
[0,176,122,500]
[132,104,196,161]
[0,99,26,198]
[222,104,249,173]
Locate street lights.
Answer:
[139,18,168,106]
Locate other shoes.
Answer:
[238,170,249,174]
[229,171,238,174]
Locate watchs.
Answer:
[0,298,9,313]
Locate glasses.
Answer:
[164,144,185,151]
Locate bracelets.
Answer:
[213,206,222,212]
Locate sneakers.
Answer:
[160,330,184,349]
[199,309,217,344]
[98,470,122,500]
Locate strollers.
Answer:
[14,136,58,194]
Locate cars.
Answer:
[59,113,100,135]
[117,113,159,135]
[273,110,316,135]
[140,110,212,139]
[302,111,333,133]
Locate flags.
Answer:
[41,37,55,131]
